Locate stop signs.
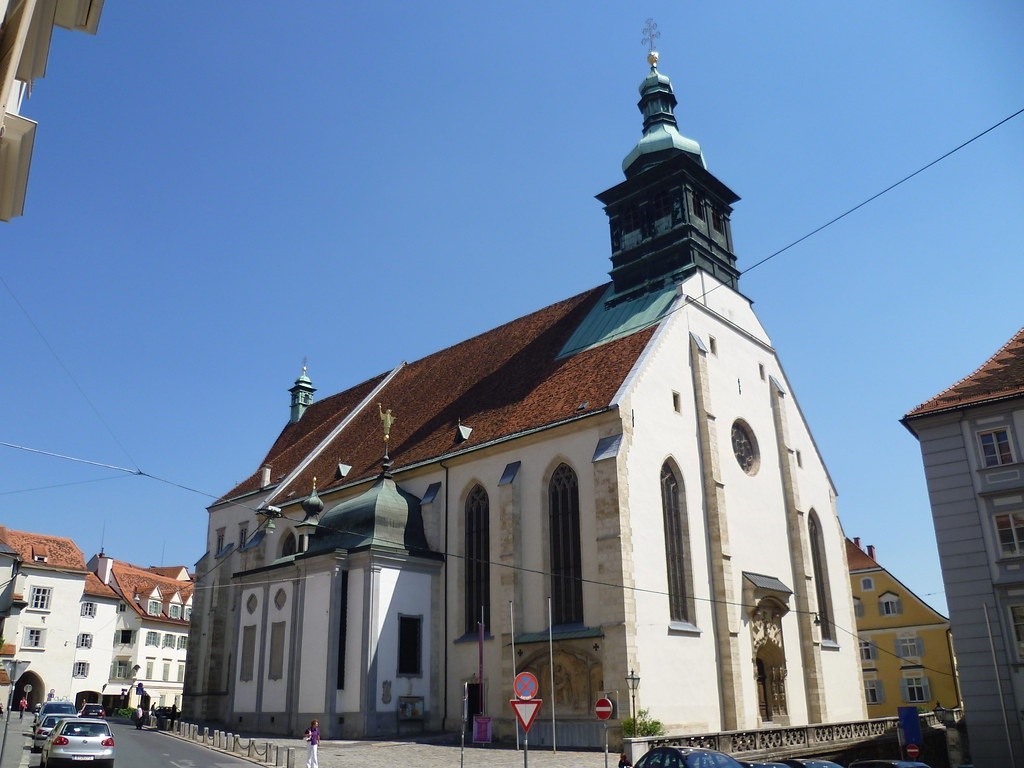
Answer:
[906,744,919,757]
[595,698,613,720]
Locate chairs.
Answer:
[47,719,55,725]
[65,726,75,733]
[94,727,103,734]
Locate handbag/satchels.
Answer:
[303,728,312,741]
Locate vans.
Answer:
[849,758,932,768]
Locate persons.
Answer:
[304,719,321,768]
[135,702,176,730]
[81,699,86,707]
[19,697,28,719]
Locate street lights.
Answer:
[625,669,640,737]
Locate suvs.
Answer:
[34,700,76,724]
[78,702,105,719]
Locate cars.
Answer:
[739,759,844,768]
[631,746,744,768]
[33,713,79,753]
[40,718,115,768]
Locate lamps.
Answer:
[933,699,966,733]
[813,616,820,626]
